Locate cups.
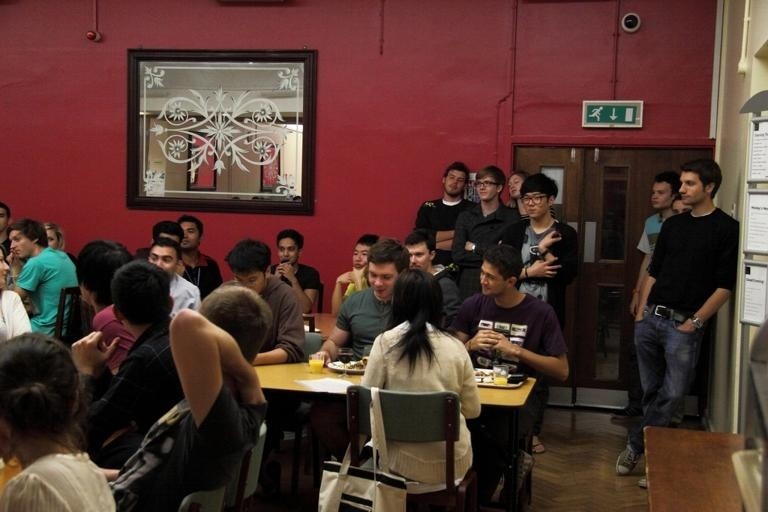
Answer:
[308,353,325,374]
[494,364,509,385]
[301,313,316,333]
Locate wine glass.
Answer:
[361,347,373,365]
[337,348,354,379]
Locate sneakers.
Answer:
[606,402,645,417]
[615,431,641,476]
[637,477,647,488]
[531,435,546,455]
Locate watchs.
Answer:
[688,315,705,331]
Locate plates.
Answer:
[327,360,366,375]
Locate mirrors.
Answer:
[261,143,280,192]
[186,130,217,191]
[126,47,318,216]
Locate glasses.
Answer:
[520,194,549,206]
[473,179,498,188]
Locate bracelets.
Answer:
[631,288,641,294]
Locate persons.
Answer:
[1,216,569,511]
[670,197,695,216]
[612,172,682,422]
[614,157,742,488]
[404,161,582,355]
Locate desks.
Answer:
[642,425,744,512]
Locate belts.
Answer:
[647,300,688,324]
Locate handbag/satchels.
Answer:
[315,384,410,512]
